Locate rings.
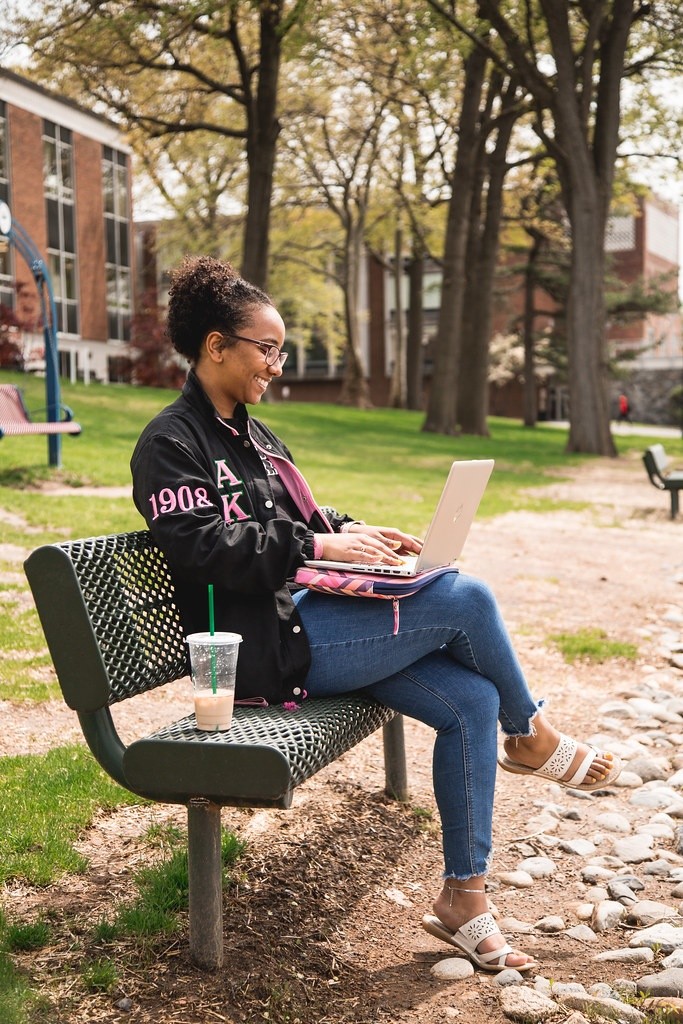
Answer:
[361,545,367,551]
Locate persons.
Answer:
[130,256,624,972]
[617,391,632,423]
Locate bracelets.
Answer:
[342,520,366,533]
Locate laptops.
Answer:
[303,458,496,578]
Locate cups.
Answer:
[185,631,244,731]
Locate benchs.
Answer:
[23,525,410,968]
[643,442,683,520]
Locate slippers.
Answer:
[497,732,622,791]
[421,912,536,971]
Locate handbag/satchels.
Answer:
[294,566,460,636]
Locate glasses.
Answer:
[221,333,288,368]
